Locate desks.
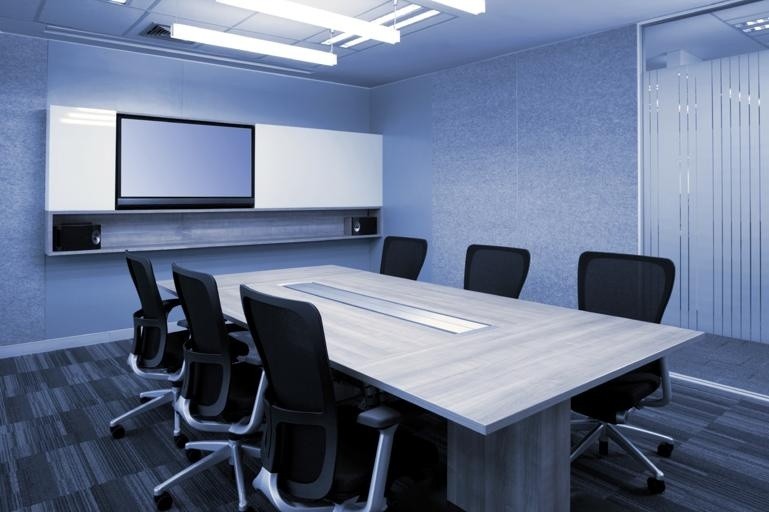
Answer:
[152,260,705,512]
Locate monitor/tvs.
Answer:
[116,111,256,212]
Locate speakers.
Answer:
[52,222,102,251]
[345,215,381,235]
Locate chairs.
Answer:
[108,250,269,511]
[463,244,531,300]
[570,251,676,493]
[239,284,404,512]
[380,235,428,280]
